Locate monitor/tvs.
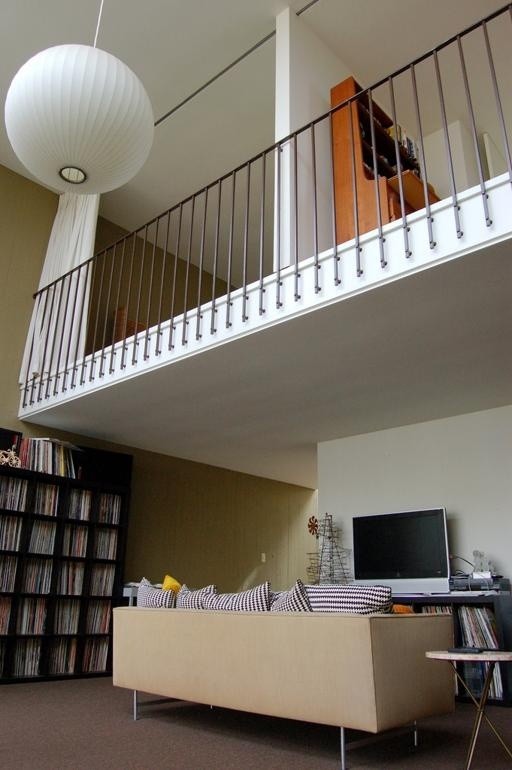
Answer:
[350,507,450,597]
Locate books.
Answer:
[422,605,504,698]
[0,437,121,677]
[389,124,417,161]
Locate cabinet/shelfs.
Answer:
[0,429,133,682]
[330,76,440,246]
[389,590,512,708]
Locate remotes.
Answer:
[447,648,483,653]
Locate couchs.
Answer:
[111,606,455,770]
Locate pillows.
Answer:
[136,574,395,613]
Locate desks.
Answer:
[122,586,139,606]
[424,651,512,770]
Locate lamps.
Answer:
[5,0,154,197]
[449,553,489,590]
